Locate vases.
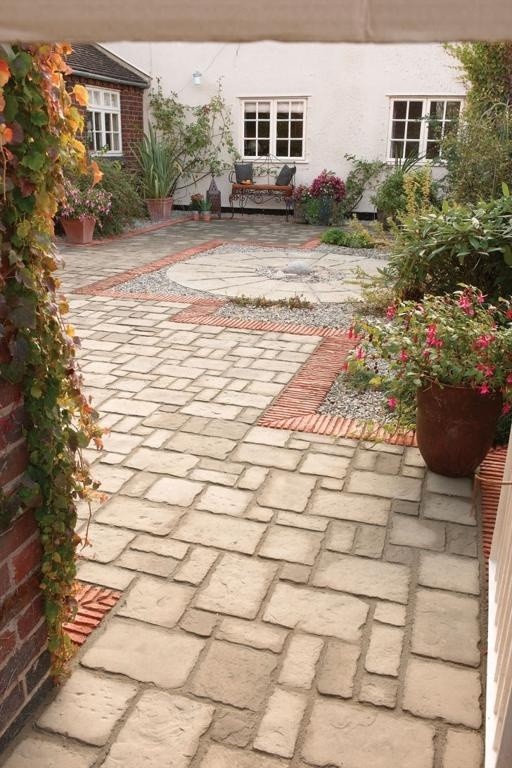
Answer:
[293,204,307,222]
[58,216,96,245]
[411,372,498,482]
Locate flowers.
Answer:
[290,168,345,205]
[332,276,511,412]
[53,180,114,221]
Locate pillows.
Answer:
[275,164,296,186]
[234,163,253,184]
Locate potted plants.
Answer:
[125,114,182,221]
[191,198,215,222]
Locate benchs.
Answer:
[226,159,298,223]
